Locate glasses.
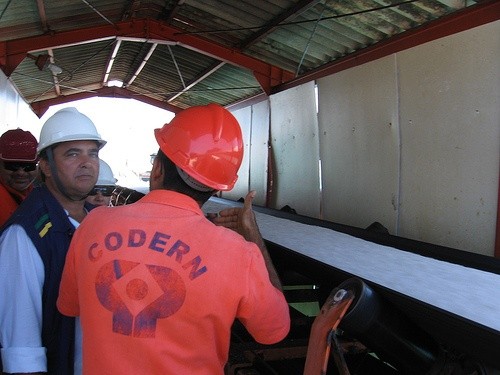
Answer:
[87,186,117,198]
[3,161,37,172]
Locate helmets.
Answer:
[0,128,38,161]
[154,103,245,192]
[37,106,108,156]
[95,158,119,186]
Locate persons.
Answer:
[88,159,118,205]
[0,106,106,375]
[0,129,38,234]
[57,102,291,375]
